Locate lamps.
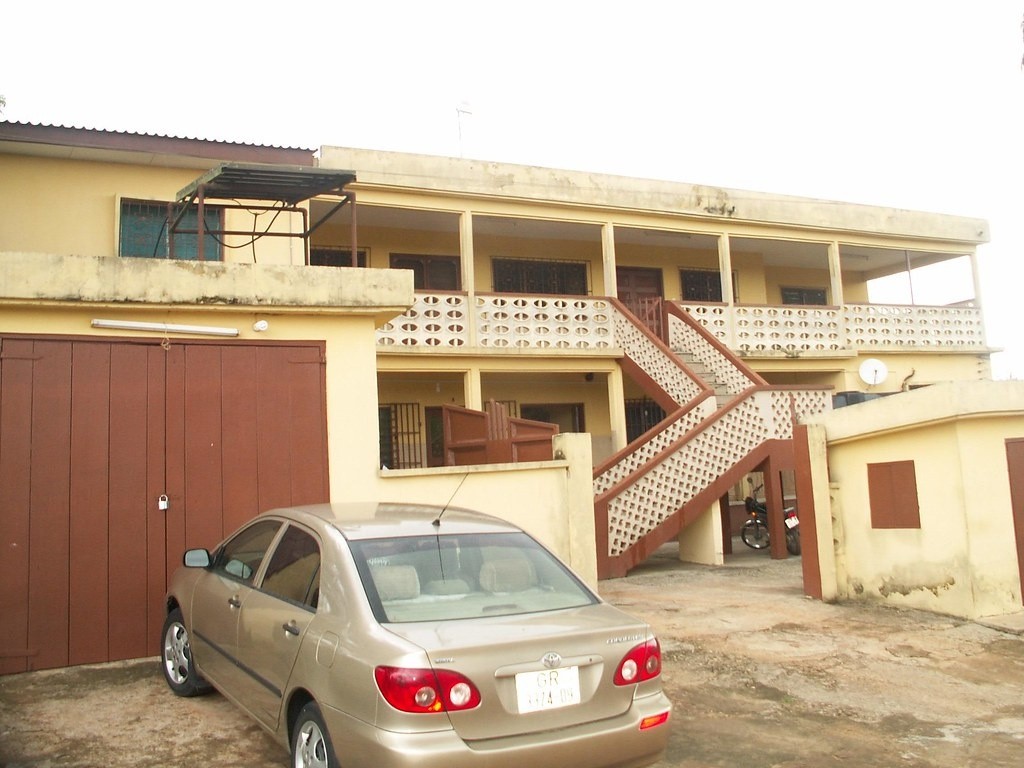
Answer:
[90,318,240,337]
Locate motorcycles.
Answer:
[740,476,802,558]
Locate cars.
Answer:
[161,498,676,768]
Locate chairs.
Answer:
[421,542,469,594]
[373,564,421,601]
[478,558,534,591]
